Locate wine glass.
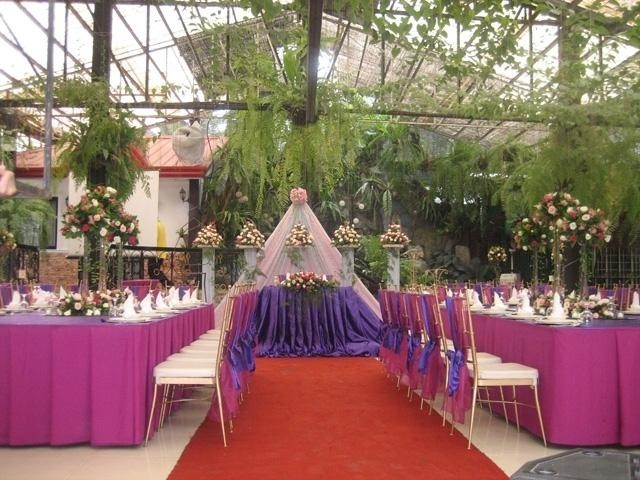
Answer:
[498,292,506,304]
[607,295,616,319]
[20,293,30,308]
[580,304,593,328]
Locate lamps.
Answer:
[179,185,190,203]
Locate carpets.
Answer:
[168,355,510,480]
[510,447,640,480]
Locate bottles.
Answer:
[273,274,279,286]
[31,285,41,304]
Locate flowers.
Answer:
[191,220,411,249]
[0,228,18,258]
[58,186,140,250]
[511,177,615,260]
[273,272,340,312]
[488,244,507,263]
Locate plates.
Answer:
[470,306,573,326]
[107,302,203,323]
[1,305,38,316]
[622,310,640,315]
